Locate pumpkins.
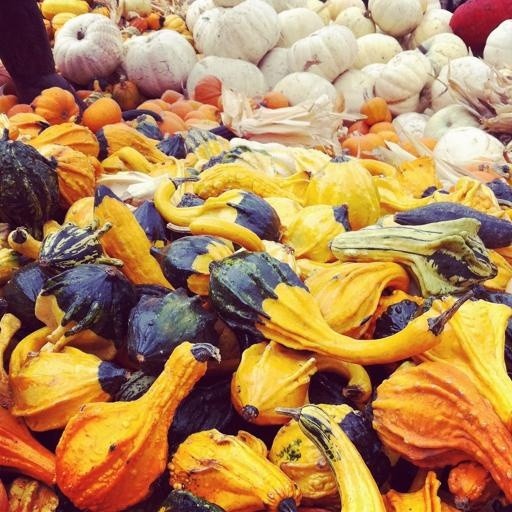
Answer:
[0,0,512,512]
